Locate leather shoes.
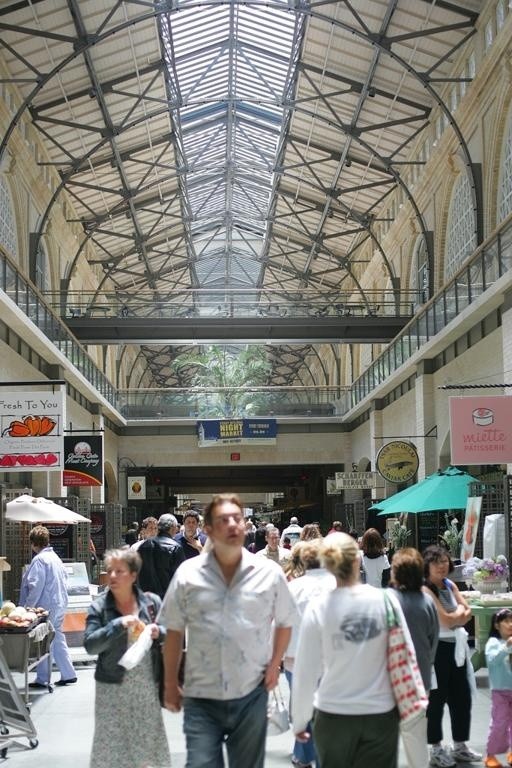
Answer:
[55,677,78,686]
[29,682,47,689]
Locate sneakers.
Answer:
[427,745,512,768]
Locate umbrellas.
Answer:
[367,469,444,547]
[5,495,92,564]
[376,466,495,544]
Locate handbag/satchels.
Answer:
[151,643,164,682]
[266,684,290,737]
[386,624,429,734]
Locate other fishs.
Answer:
[384,460,415,470]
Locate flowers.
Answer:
[437,512,464,548]
[461,554,509,591]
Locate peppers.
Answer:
[3,414,57,437]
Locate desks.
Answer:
[459,590,512,689]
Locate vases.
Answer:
[450,544,458,558]
[471,579,503,595]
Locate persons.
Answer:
[483,608,512,768]
[19,526,77,689]
[83,494,483,767]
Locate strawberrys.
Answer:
[0,453,57,466]
